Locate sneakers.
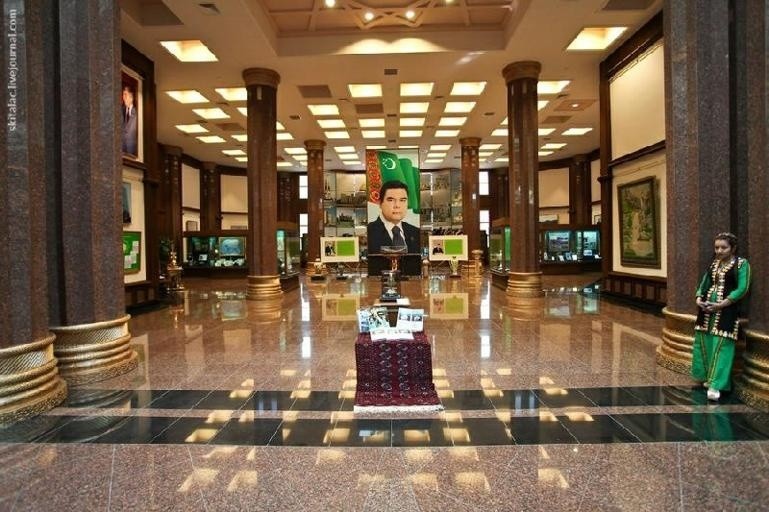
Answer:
[707,389,720,402]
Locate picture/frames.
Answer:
[122,231,142,276]
[117,68,141,160]
[120,179,132,228]
[616,174,661,270]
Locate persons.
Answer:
[433,242,443,255]
[118,82,140,156]
[326,242,336,255]
[690,232,752,402]
[368,179,421,276]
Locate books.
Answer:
[355,304,426,342]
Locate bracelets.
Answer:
[717,303,721,310]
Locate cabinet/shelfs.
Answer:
[178,227,250,280]
[366,295,413,328]
[275,221,302,291]
[353,328,440,406]
[487,223,511,291]
[537,223,605,275]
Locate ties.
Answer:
[392,226,405,248]
